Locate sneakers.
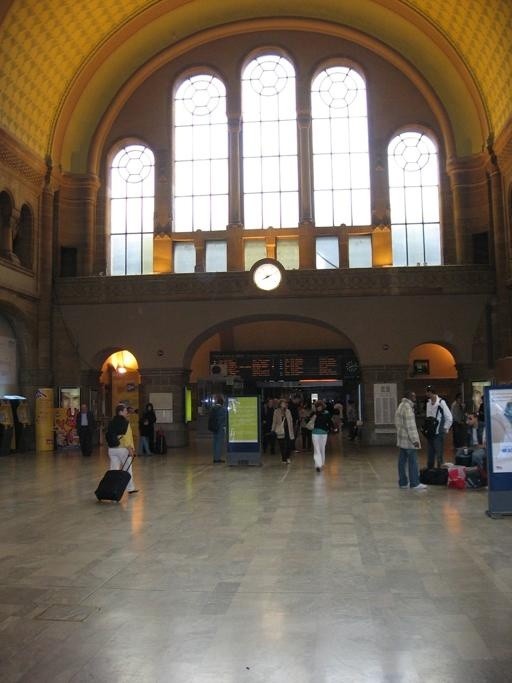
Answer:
[316,466,324,472]
[213,459,225,463]
[138,453,154,456]
[292,447,313,453]
[400,482,428,489]
[281,458,291,464]
[129,490,139,494]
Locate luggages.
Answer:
[94,454,137,501]
[156,424,167,454]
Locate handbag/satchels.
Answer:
[275,417,286,439]
[302,414,317,431]
[419,466,448,486]
[420,415,439,438]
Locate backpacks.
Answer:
[105,423,123,448]
[207,407,222,434]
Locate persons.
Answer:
[420,383,486,474]
[393,389,428,490]
[260,392,357,472]
[76,402,95,458]
[104,403,140,496]
[126,402,156,456]
[207,397,229,464]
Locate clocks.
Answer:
[249,258,288,293]
